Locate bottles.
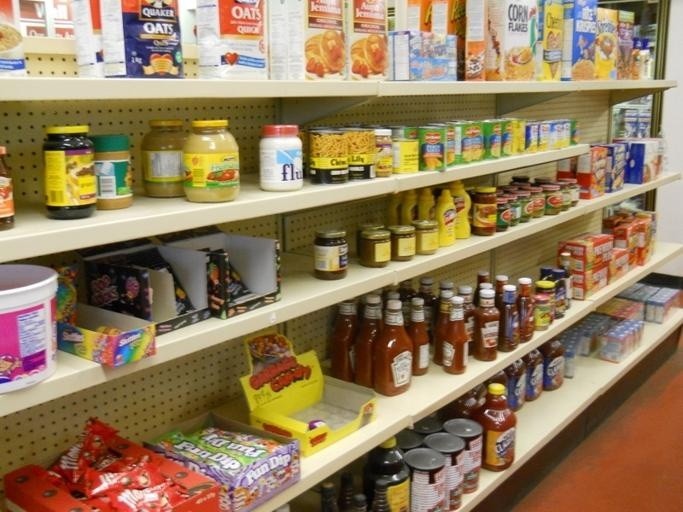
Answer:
[631,36,653,80]
[0,146,15,231]
[259,124,304,191]
[326,254,574,410]
[392,178,473,247]
[321,383,518,512]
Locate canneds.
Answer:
[308,117,581,331]
[141,120,186,198]
[41,125,97,220]
[0,146,15,231]
[90,134,135,210]
[184,120,241,202]
[395,416,484,512]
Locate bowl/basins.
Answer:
[594,31,617,77]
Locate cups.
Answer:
[0,261,59,393]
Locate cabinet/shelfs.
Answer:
[0,0,410,512]
[378,77,683,512]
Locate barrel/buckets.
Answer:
[442,337,564,471]
[0,264,60,393]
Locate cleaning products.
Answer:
[386,182,471,245]
